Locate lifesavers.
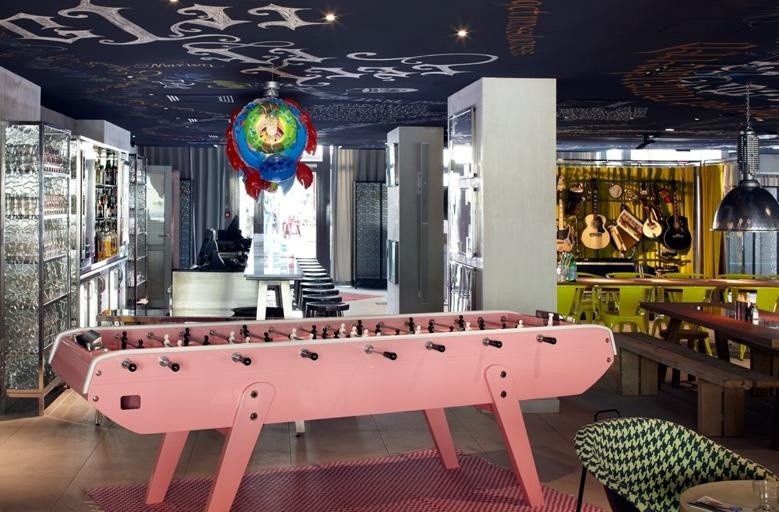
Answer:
[227,96,317,199]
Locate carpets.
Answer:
[81,444,607,512]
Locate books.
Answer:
[686,496,743,512]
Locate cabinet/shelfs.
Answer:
[0,119,151,415]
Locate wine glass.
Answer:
[752,479,779,511]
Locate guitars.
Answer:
[556,168,691,252]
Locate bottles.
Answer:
[95,149,119,261]
[751,303,759,321]
[561,250,578,283]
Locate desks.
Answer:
[241,231,305,321]
[573,275,779,440]
[677,480,779,512]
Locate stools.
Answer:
[292,256,349,318]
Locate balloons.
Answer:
[226,97,317,200]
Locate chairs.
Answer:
[563,409,778,511]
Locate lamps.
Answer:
[711,79,779,232]
